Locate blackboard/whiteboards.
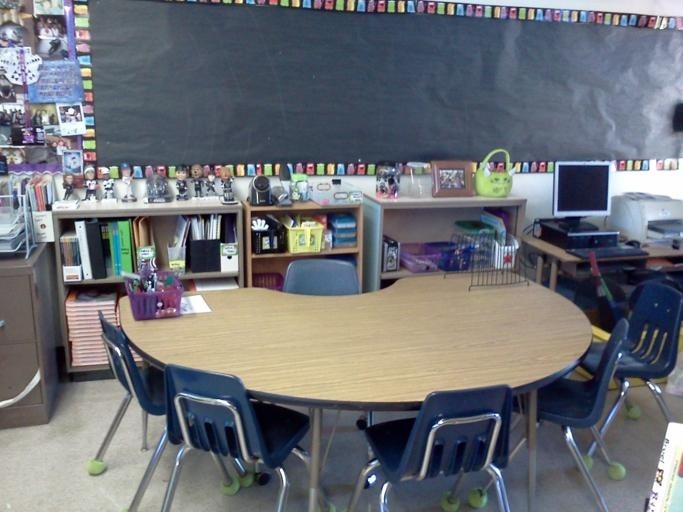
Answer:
[65,1,683,181]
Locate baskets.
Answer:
[253,216,323,254]
[400,241,470,272]
[125,272,183,320]
[252,272,283,290]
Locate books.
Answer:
[1,177,54,252]
[646,421,682,512]
[60,213,240,368]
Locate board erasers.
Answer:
[673,102,683,134]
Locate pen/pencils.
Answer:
[127,271,181,319]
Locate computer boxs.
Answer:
[540,220,620,249]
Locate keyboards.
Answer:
[564,247,649,259]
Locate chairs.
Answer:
[483,317,630,512]
[162,364,310,511]
[349,384,512,512]
[89,310,165,511]
[577,279,682,471]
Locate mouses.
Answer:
[625,240,640,247]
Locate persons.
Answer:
[62,164,236,202]
[439,169,465,189]
[0,0,88,188]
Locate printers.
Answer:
[603,194,683,243]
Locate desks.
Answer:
[522,235,683,292]
[117,270,593,512]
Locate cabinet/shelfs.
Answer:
[0,237,61,430]
[363,194,528,287]
[51,192,244,381]
[244,201,363,292]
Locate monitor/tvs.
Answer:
[554,162,612,231]
